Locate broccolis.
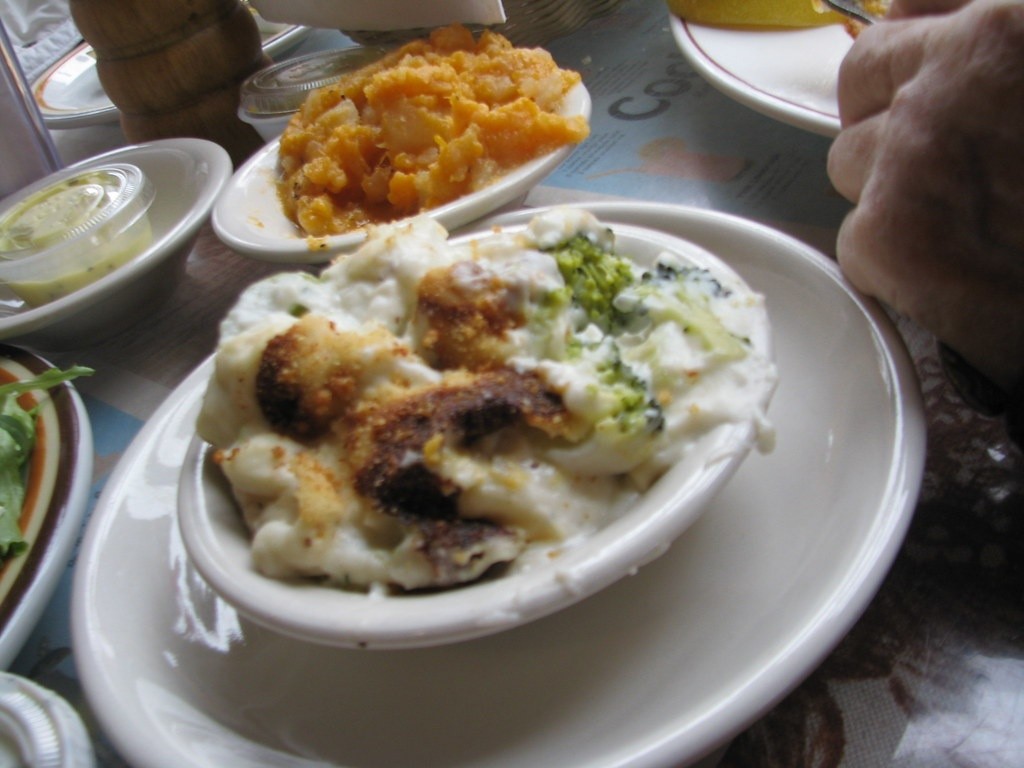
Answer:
[514,224,748,470]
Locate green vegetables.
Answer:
[0,364,95,570]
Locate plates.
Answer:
[31,0,317,127]
[73,201,926,768]
[0,342,93,672]
[668,1,856,138]
[211,70,592,265]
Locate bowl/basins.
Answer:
[238,44,389,142]
[0,137,234,347]
[175,223,769,651]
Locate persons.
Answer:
[825,0,1022,405]
